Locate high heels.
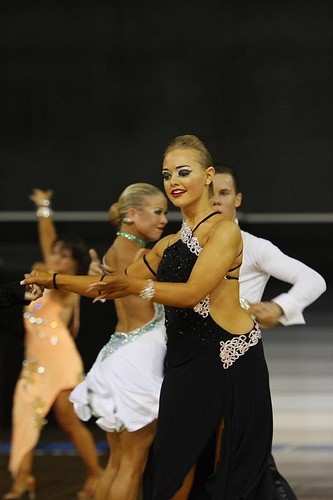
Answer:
[3,475,37,500]
[77,469,104,500]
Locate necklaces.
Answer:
[116,231,146,248]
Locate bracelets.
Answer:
[35,201,53,217]
[53,273,58,289]
[139,279,155,303]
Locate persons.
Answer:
[0,135,326,500]
